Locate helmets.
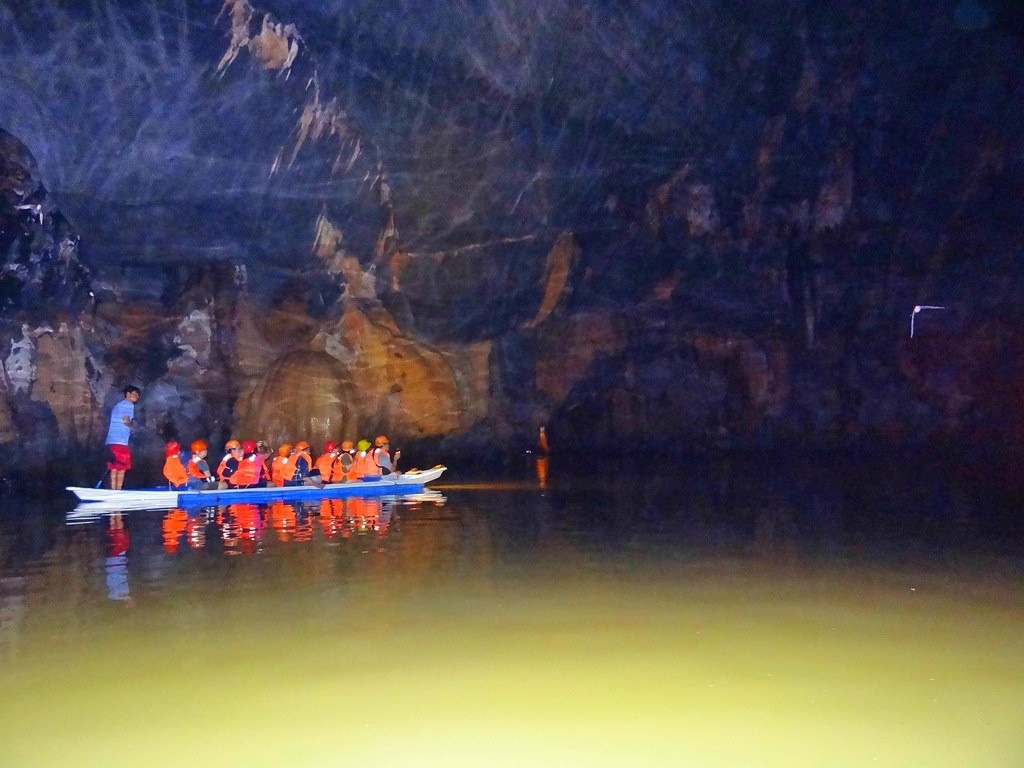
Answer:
[243,439,256,454]
[225,440,241,449]
[278,444,293,456]
[341,441,354,452]
[191,440,208,453]
[296,441,312,451]
[325,441,337,452]
[358,439,371,451]
[166,441,181,455]
[375,436,389,446]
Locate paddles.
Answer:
[92,417,136,488]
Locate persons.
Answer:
[105,385,140,489]
[163,435,402,491]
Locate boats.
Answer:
[64,465,448,501]
